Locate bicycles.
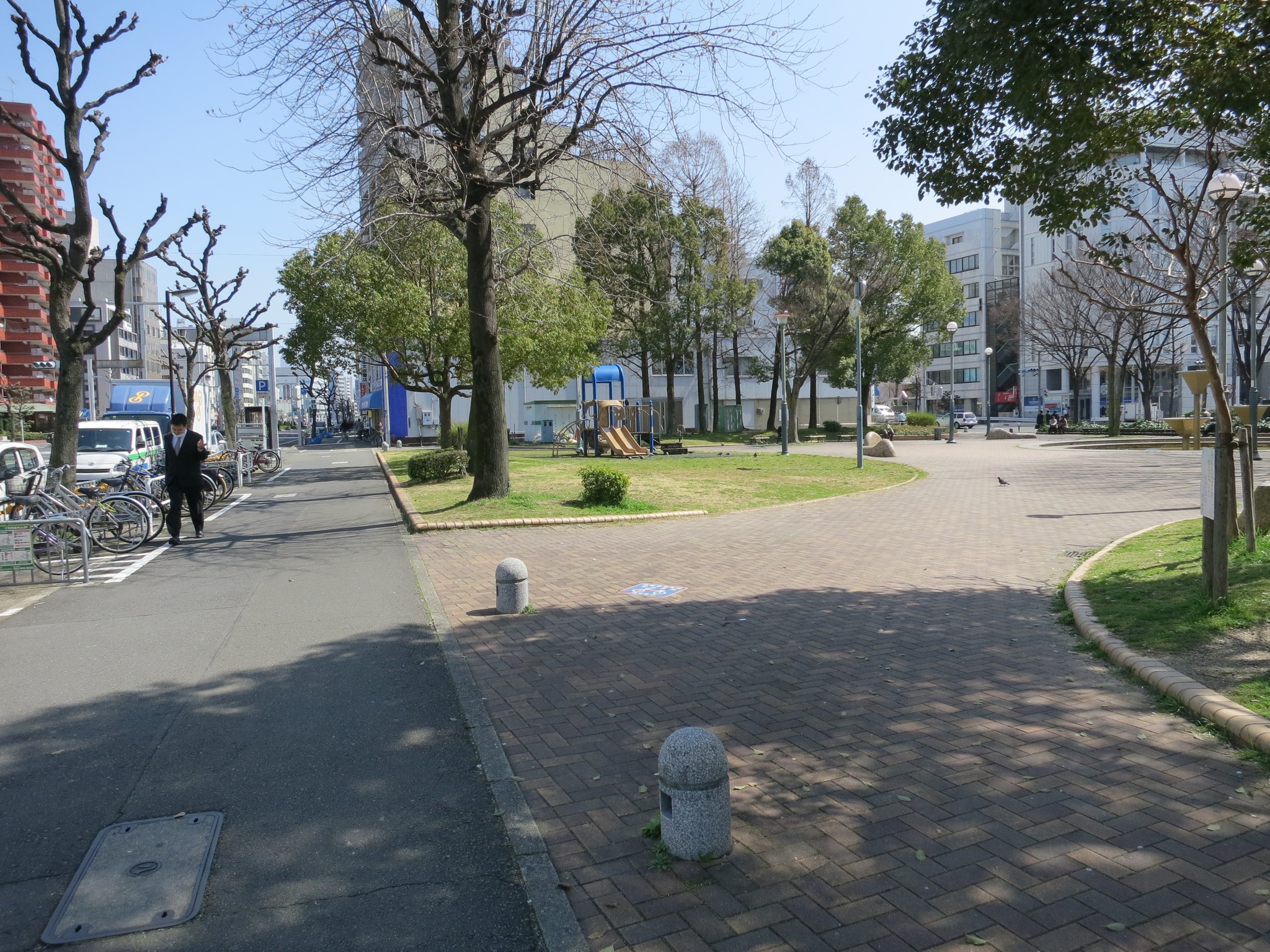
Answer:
[0,448,234,575]
[340,428,349,441]
[354,428,397,447]
[300,431,309,445]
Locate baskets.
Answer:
[226,440,238,451]
[151,453,165,465]
[119,456,142,473]
[29,467,59,491]
[4,467,37,496]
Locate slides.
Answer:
[600,425,647,456]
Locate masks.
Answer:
[171,429,187,438]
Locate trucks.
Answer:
[102,379,212,452]
[316,421,327,433]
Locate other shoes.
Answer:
[377,447,380,448]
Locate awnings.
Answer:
[359,390,382,411]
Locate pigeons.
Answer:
[717,450,723,456]
[580,448,583,454]
[721,443,725,446]
[627,456,632,460]
[639,454,645,461]
[574,449,581,454]
[688,451,694,454]
[665,451,668,455]
[752,452,757,458]
[725,451,730,456]
[648,452,655,457]
[997,477,1010,486]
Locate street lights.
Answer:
[774,314,791,456]
[853,273,867,469]
[946,321,958,443]
[984,347,993,436]
[1205,172,1242,406]
[1242,259,1264,461]
[165,288,199,417]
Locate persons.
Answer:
[883,420,894,441]
[897,410,906,426]
[164,413,208,545]
[340,418,384,448]
[1036,406,1071,435]
[1101,406,1106,417]
[1202,408,1214,417]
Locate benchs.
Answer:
[879,434,892,440]
[804,435,826,444]
[1120,428,1142,434]
[1049,428,1070,434]
[1153,429,1174,437]
[775,438,782,443]
[1082,428,1105,435]
[837,435,857,442]
[748,437,770,444]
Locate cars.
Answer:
[894,411,907,417]
[363,421,370,431]
[0,441,48,522]
[46,432,55,444]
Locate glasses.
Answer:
[171,425,187,432]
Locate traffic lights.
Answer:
[308,408,313,418]
[311,399,316,409]
[33,361,56,369]
[1044,390,1048,397]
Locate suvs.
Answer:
[935,412,978,429]
[210,430,228,458]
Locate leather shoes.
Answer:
[168,536,181,545]
[196,531,204,538]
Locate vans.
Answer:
[871,404,896,422]
[75,419,164,493]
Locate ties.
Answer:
[174,437,181,456]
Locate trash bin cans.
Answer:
[934,428,947,440]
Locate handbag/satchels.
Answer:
[1035,424,1040,429]
[360,428,364,431]
